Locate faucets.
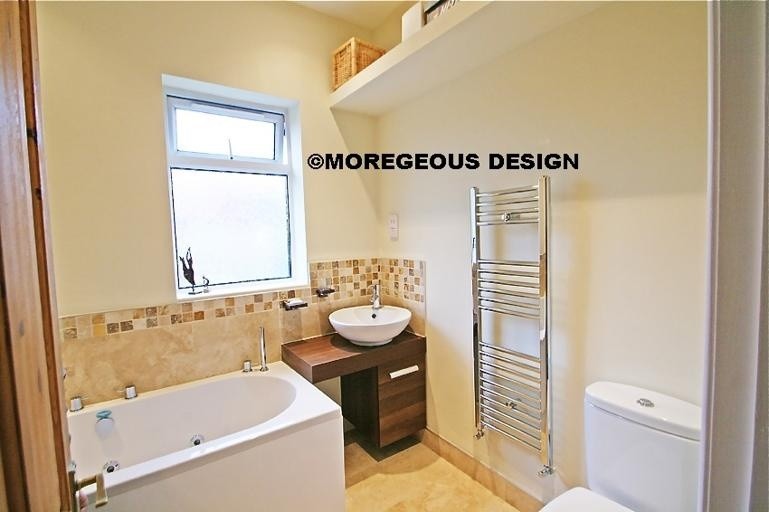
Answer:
[372,283,383,309]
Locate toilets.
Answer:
[538,380,702,512]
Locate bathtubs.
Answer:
[65,359,347,512]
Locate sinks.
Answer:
[328,305,412,346]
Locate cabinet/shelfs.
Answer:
[341,354,426,449]
